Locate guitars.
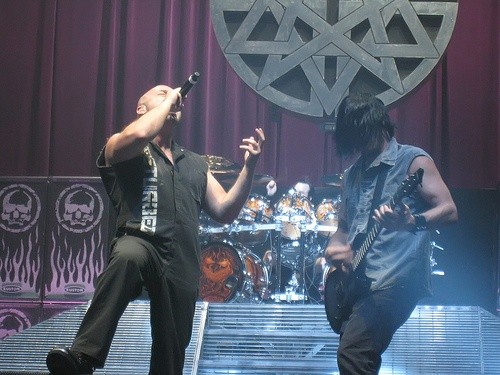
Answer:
[323,168,425,334]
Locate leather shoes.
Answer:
[46,347,94,375]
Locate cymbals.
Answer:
[221,173,274,184]
[200,155,242,173]
[325,182,342,186]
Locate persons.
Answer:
[46,72,266,375]
[269,182,327,304]
[324,93,459,375]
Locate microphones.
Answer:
[176,72,200,107]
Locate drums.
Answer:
[274,236,322,270]
[273,193,316,240]
[197,238,272,303]
[229,193,273,247]
[316,199,338,238]
